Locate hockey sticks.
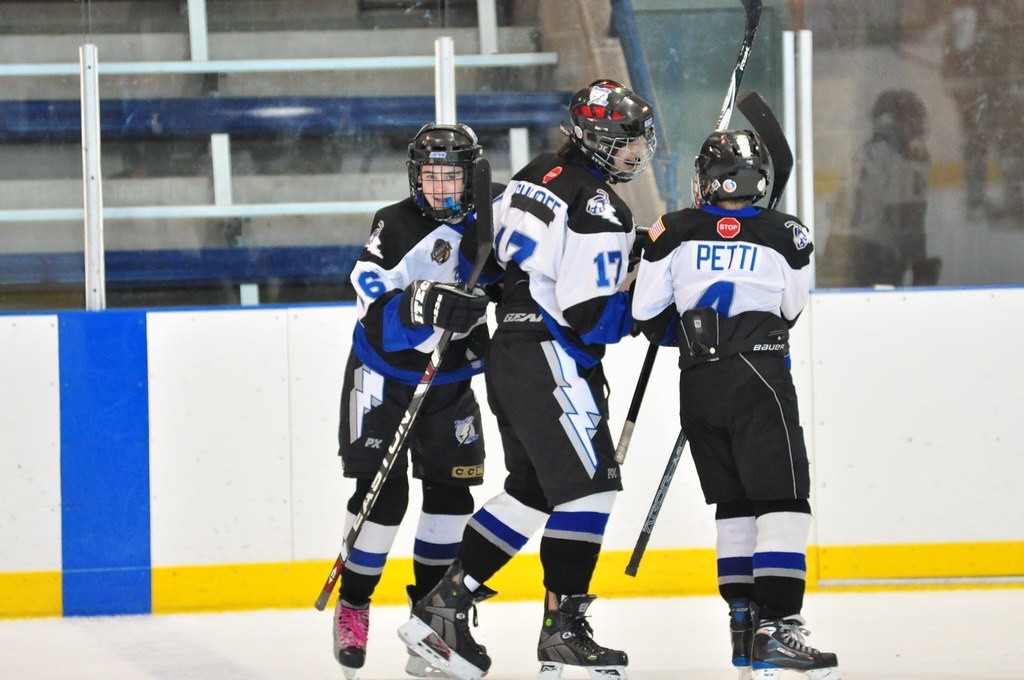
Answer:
[307,153,502,614]
[618,81,800,587]
[609,0,765,476]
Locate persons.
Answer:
[332,122,499,679]
[847,0,1024,289]
[395,78,659,678]
[631,128,840,680]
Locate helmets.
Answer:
[407,122,483,188]
[560,76,654,168]
[694,128,770,203]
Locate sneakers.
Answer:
[748,614,841,680]
[538,593,629,680]
[397,557,491,680]
[332,595,371,680]
[728,597,752,680]
[405,584,449,678]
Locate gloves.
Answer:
[398,279,490,334]
